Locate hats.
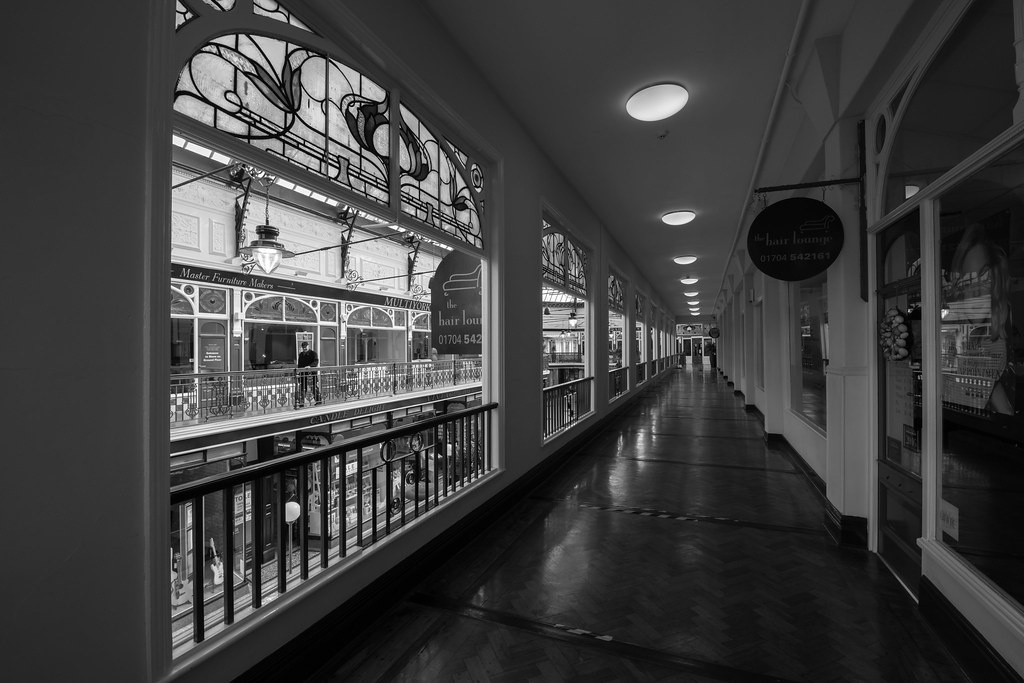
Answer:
[302,342,308,347]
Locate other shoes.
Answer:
[294,405,304,407]
[315,402,322,405]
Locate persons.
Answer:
[947,343,957,368]
[939,222,1016,416]
[293,342,321,408]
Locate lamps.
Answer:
[689,308,700,312]
[687,301,699,305]
[661,211,696,226]
[172,162,296,276]
[625,83,689,122]
[683,292,698,297]
[674,257,697,265]
[681,279,699,285]
[568,312,584,326]
[691,313,701,316]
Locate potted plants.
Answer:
[706,342,717,368]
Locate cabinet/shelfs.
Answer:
[309,463,374,536]
